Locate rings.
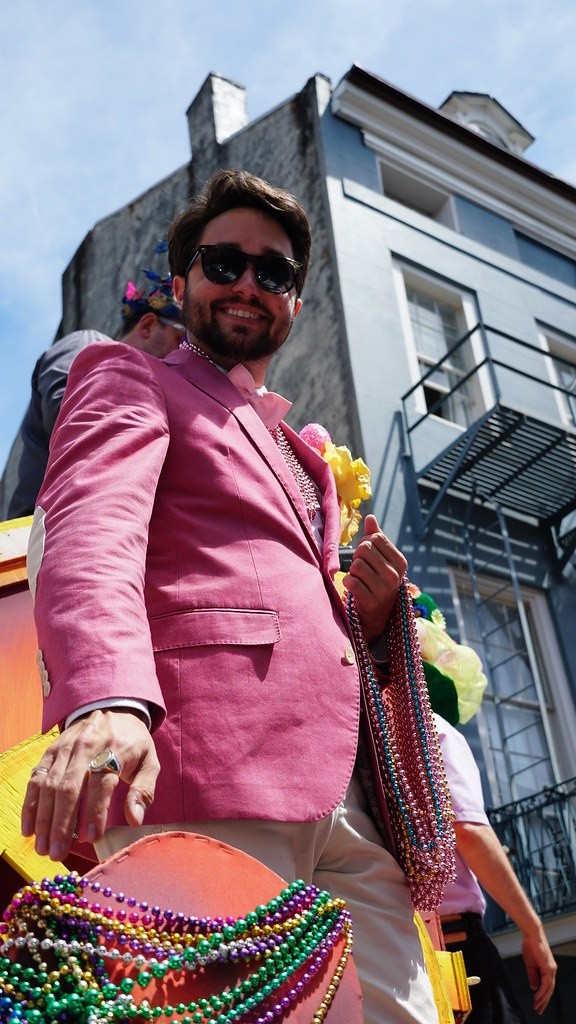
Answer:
[89,750,122,777]
[31,767,48,774]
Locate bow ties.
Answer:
[226,363,293,431]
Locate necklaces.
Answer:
[342,571,455,906]
[177,341,321,517]
[0,879,354,1024]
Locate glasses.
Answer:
[183,244,303,297]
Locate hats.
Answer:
[406,580,486,727]
[120,269,185,327]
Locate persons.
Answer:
[21,168,433,1024]
[6,285,189,520]
[431,709,557,1024]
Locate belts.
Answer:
[442,914,485,936]
